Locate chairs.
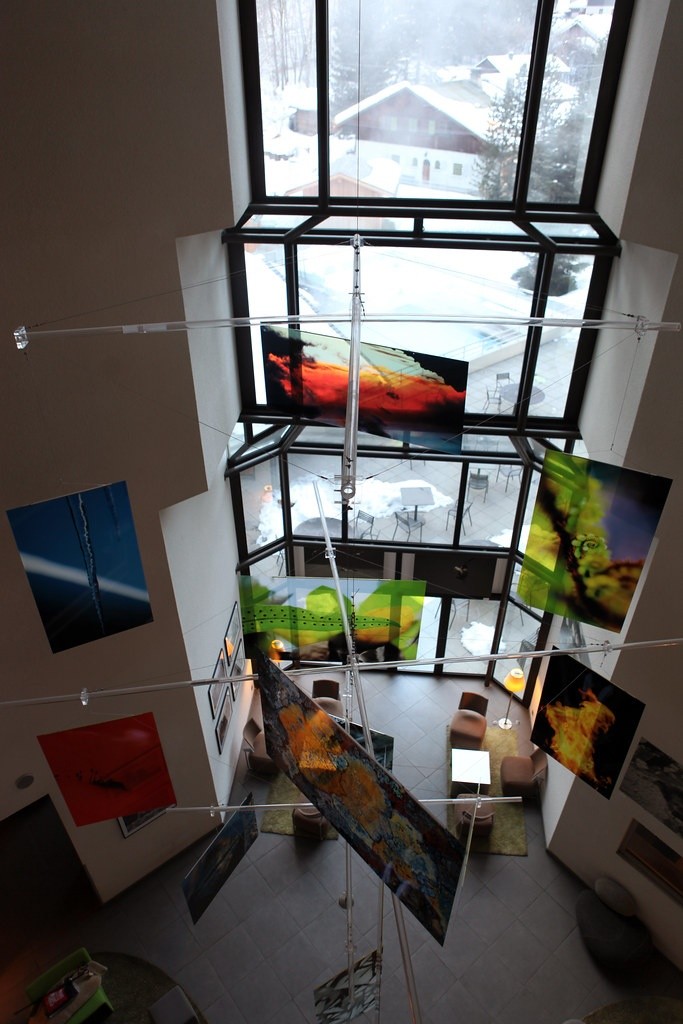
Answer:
[275,533,285,576]
[432,597,471,630]
[444,499,475,536]
[481,372,515,414]
[391,512,426,543]
[467,473,490,504]
[495,464,524,493]
[509,581,531,627]
[349,510,375,540]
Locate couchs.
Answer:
[292,792,331,847]
[499,749,547,796]
[147,984,200,1024]
[450,691,491,750]
[455,790,496,830]
[243,718,280,775]
[312,681,344,718]
[25,947,117,1024]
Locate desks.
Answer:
[502,381,546,414]
[400,486,436,529]
[295,517,351,540]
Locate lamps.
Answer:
[267,639,288,667]
[499,666,526,730]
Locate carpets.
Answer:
[444,726,528,857]
[88,950,209,1024]
[260,771,339,841]
[582,996,683,1024]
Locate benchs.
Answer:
[574,890,654,968]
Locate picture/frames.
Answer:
[117,803,178,839]
[229,638,246,702]
[207,647,229,720]
[223,600,242,668]
[215,685,235,756]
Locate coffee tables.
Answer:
[448,747,493,796]
